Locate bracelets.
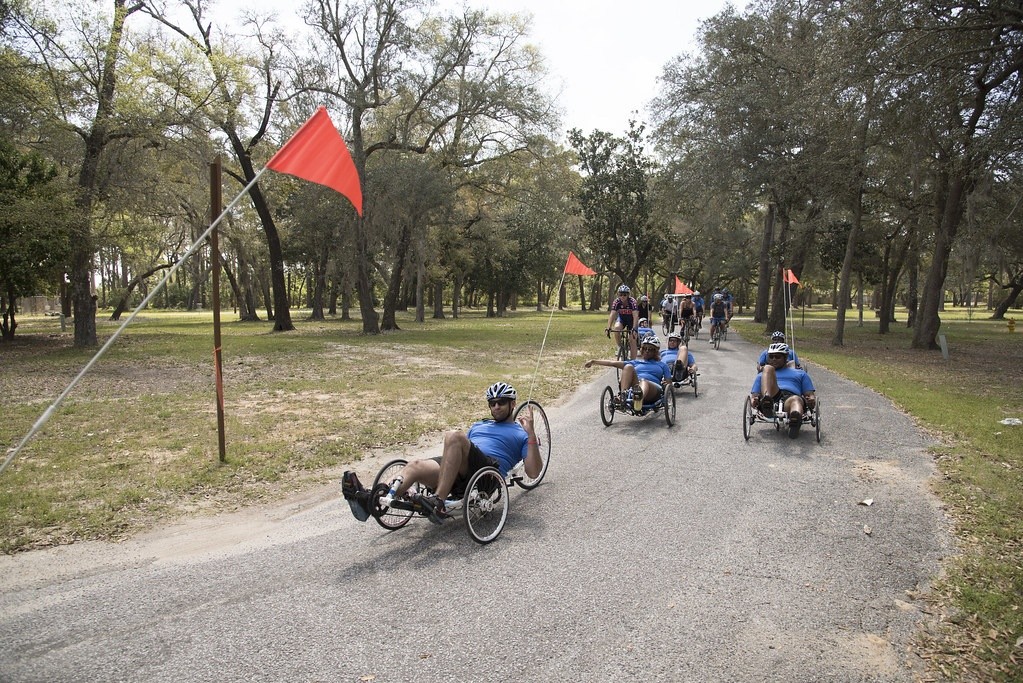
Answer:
[527,431,535,434]
[527,441,537,444]
[528,436,536,438]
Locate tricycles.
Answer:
[600,380,680,428]
[660,365,701,398]
[742,394,821,443]
[756,361,809,374]
[341,400,551,544]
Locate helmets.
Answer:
[768,342,789,354]
[486,381,516,401]
[664,287,729,300]
[771,331,785,340]
[617,285,630,293]
[638,318,647,325]
[668,332,682,339]
[641,336,660,349]
[641,296,647,301]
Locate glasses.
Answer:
[774,338,781,341]
[643,347,654,351]
[620,294,627,296]
[489,398,507,407]
[642,301,647,303]
[670,339,678,342]
[769,354,783,359]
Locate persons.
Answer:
[755,331,803,373]
[750,341,817,439]
[659,294,677,333]
[678,294,698,340]
[708,287,733,343]
[656,332,697,381]
[606,285,639,360]
[691,290,705,328]
[638,294,653,326]
[585,335,672,412]
[636,318,655,347]
[341,381,543,525]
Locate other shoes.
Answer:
[342,472,372,522]
[788,411,802,439]
[615,351,623,357]
[675,360,684,381]
[709,338,714,343]
[411,493,447,520]
[632,384,643,411]
[761,395,773,419]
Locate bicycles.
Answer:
[605,328,634,384]
[658,311,730,351]
